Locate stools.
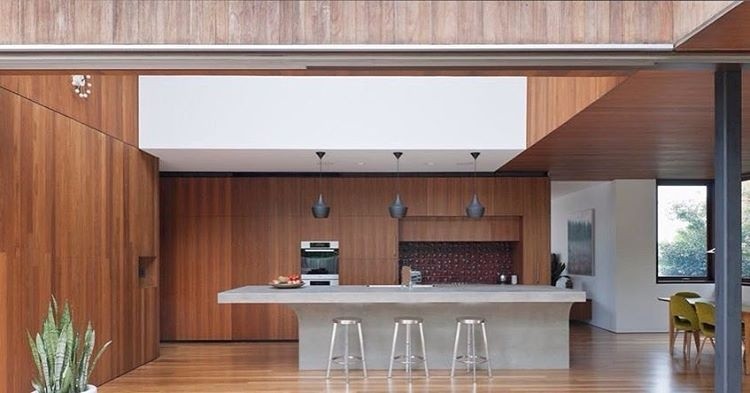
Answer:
[389,316,430,378]
[451,316,492,377]
[326,317,367,379]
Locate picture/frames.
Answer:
[567,208,595,276]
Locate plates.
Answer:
[269,282,305,288]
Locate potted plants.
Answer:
[26,295,112,393]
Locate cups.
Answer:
[511,275,517,285]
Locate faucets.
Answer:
[407,263,413,289]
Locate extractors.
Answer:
[300,240,339,253]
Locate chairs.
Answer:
[672,291,717,372]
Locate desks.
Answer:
[657,297,750,376]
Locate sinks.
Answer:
[367,283,434,288]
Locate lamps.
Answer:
[388,152,408,218]
[466,152,485,218]
[312,152,330,218]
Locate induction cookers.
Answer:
[301,274,339,279]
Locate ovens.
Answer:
[302,279,339,285]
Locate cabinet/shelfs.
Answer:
[570,299,592,320]
